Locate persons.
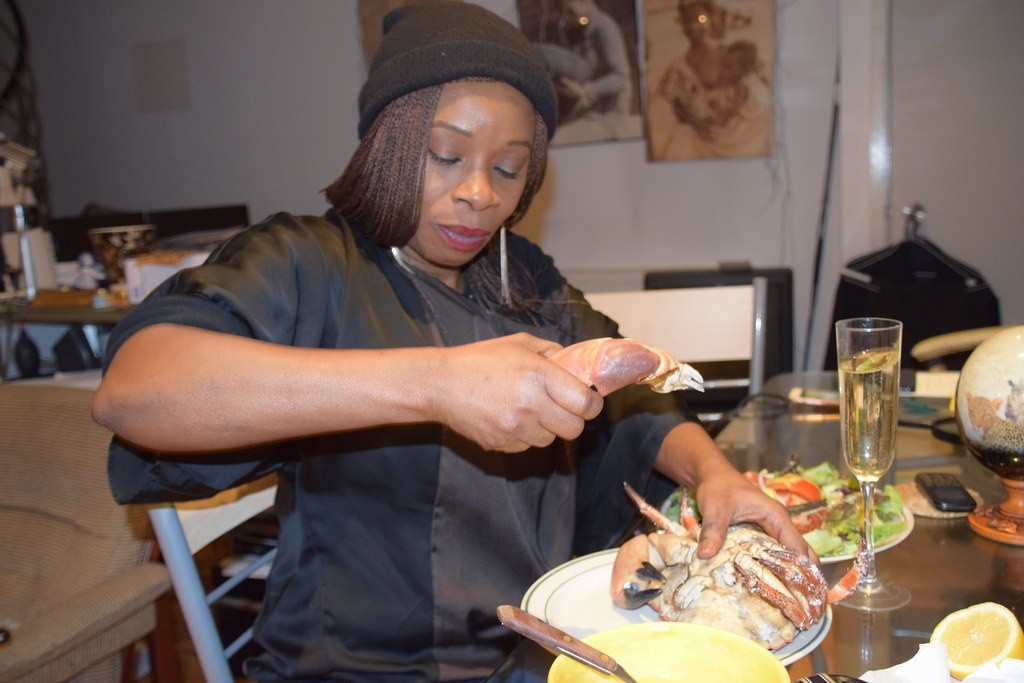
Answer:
[91,0,824,683]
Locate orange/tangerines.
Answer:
[930,602,1024,680]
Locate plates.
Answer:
[659,490,915,562]
[520,547,832,665]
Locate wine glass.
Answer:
[827,318,912,611]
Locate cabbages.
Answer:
[773,461,908,557]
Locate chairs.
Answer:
[148,472,284,683]
[584,276,767,425]
[0,382,166,683]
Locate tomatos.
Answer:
[766,474,822,503]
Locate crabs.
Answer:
[548,336,708,396]
[609,481,864,651]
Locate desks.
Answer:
[0,304,136,369]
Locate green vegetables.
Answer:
[665,489,701,521]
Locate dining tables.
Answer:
[477,371,1024,683]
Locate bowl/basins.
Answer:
[547,621,790,683]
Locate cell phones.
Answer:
[915,473,977,511]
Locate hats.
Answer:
[357,0,559,141]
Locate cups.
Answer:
[89,224,154,285]
